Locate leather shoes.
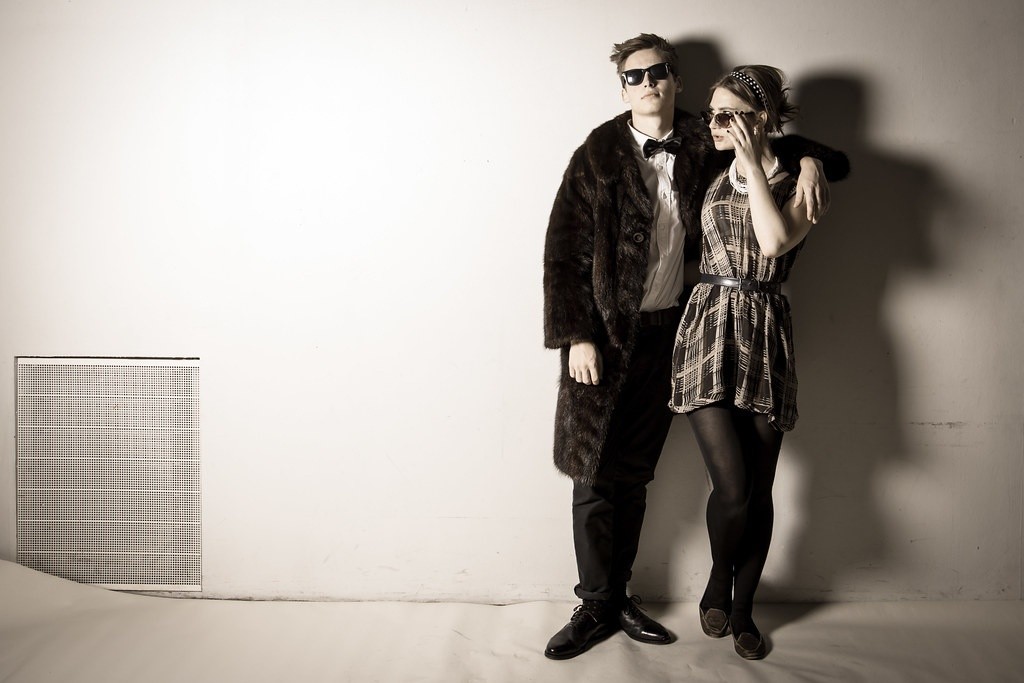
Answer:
[545,598,620,660]
[617,591,672,644]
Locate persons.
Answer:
[667,65,823,660]
[543,33,852,661]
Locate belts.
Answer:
[639,308,681,326]
[699,271,781,295]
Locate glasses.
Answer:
[700,110,755,128]
[621,61,677,86]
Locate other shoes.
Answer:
[699,605,733,638]
[729,618,766,659]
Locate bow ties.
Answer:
[643,135,683,158]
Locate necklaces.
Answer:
[728,156,782,195]
